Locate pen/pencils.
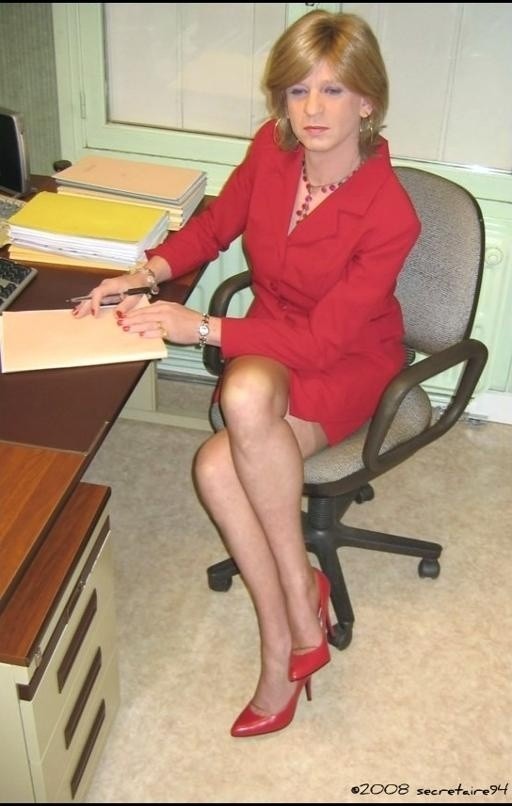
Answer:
[65,287,151,303]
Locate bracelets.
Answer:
[133,266,159,299]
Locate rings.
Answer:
[157,322,161,331]
[162,331,168,337]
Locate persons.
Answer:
[72,11,423,738]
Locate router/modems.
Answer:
[0,108,37,200]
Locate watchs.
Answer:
[198,315,210,349]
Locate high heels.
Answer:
[289,566,337,682]
[231,673,312,736]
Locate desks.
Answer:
[1,167,232,806]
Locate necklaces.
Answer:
[296,156,366,226]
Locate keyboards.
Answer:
[0,257,38,316]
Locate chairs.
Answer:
[200,163,488,659]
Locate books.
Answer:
[157,348,218,378]
[4,154,208,271]
[0,296,168,372]
